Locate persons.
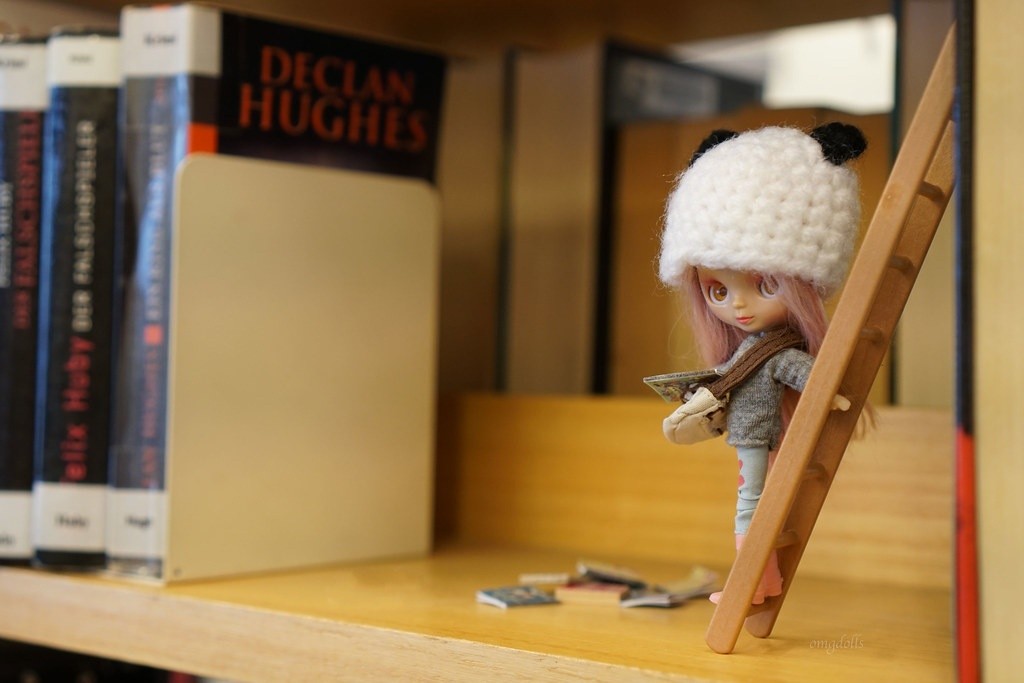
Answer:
[643,119,868,608]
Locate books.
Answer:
[478,559,719,609]
[0,0,457,577]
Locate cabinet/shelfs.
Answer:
[0,0,1024,683]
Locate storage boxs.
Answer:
[439,61,503,392]
[503,37,762,411]
[611,110,818,399]
[820,116,893,408]
